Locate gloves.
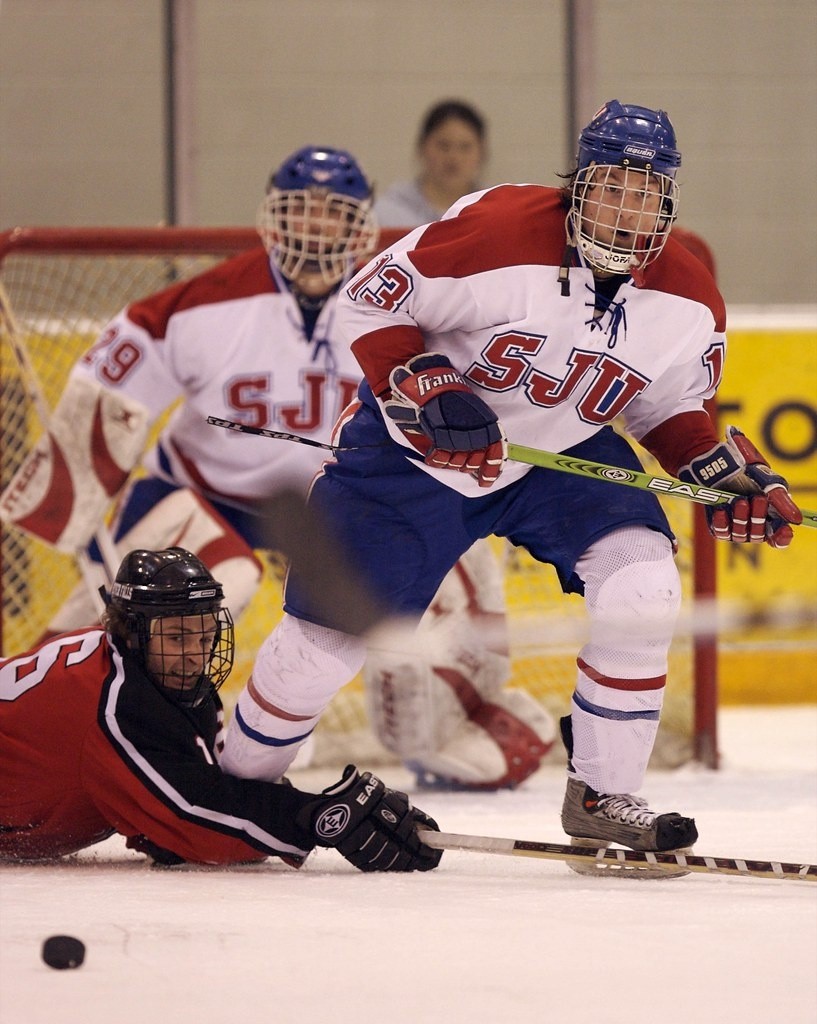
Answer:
[679,426,802,550]
[381,352,504,488]
[298,761,443,874]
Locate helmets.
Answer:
[565,99,682,275]
[107,545,236,711]
[257,145,377,287]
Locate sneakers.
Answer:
[560,774,698,880]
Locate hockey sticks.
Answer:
[507,438,816,531]
[417,826,817,886]
[0,286,125,588]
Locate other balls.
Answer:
[41,933,86,971]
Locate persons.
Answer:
[0,146,560,784]
[213,98,803,852]
[0,547,444,872]
